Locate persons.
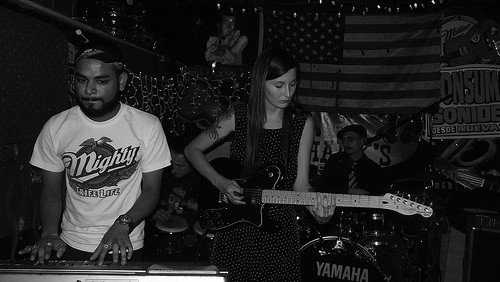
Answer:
[19,40,172,267]
[205,13,248,65]
[149,140,208,222]
[187,51,335,282]
[320,124,392,196]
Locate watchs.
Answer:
[114,214,133,234]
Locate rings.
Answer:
[58,243,66,248]
[24,246,31,252]
[33,244,39,248]
[102,244,109,250]
[45,241,53,246]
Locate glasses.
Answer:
[340,136,361,144]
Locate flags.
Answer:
[253,10,441,115]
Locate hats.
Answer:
[336,125,367,139]
[73,38,124,70]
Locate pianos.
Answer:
[0,259,231,282]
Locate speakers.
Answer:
[462,226,500,282]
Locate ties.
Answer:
[348,161,359,189]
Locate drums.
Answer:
[297,236,389,282]
[357,211,408,249]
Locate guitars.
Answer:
[196,157,434,230]
